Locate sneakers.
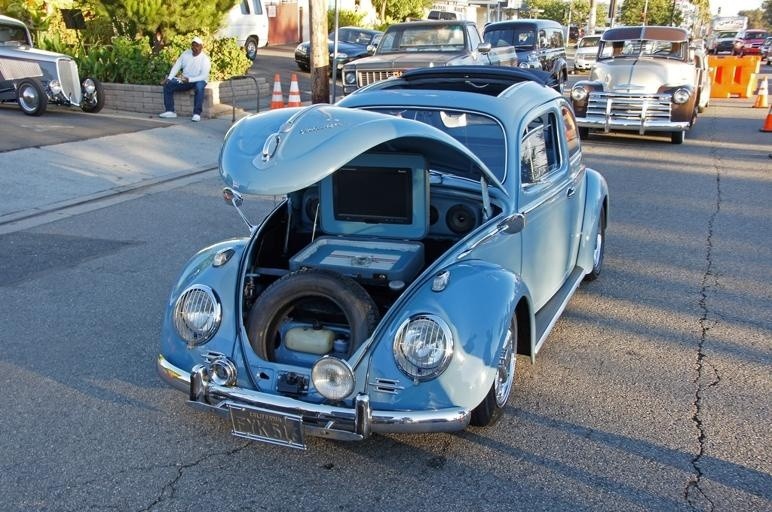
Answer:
[159,110,177,118]
[192,114,200,122]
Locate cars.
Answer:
[711,25,772,66]
[293,22,394,80]
[148,58,616,458]
[568,25,605,71]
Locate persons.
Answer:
[159,37,211,122]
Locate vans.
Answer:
[210,0,271,62]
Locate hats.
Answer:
[192,37,203,46]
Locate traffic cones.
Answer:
[268,72,284,109]
[758,102,772,133]
[287,72,304,107]
[751,74,772,108]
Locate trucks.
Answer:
[705,16,751,52]
[340,18,518,105]
[406,7,467,41]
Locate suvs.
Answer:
[573,21,705,147]
[480,17,571,95]
[0,12,109,118]
